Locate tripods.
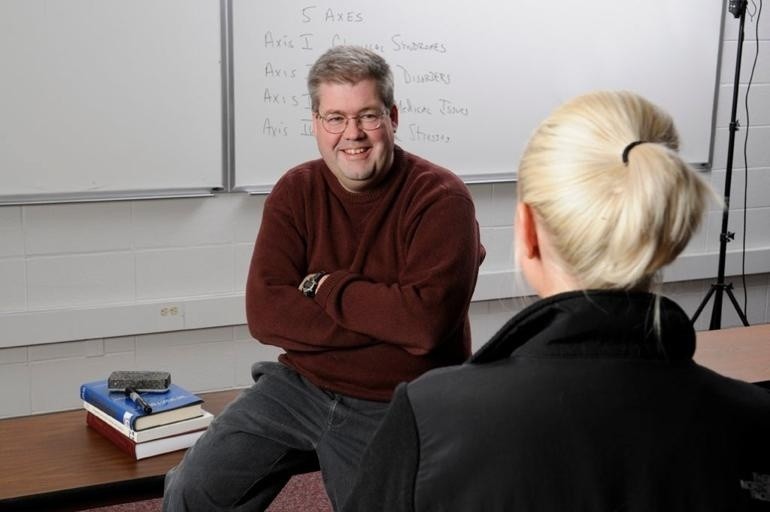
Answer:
[691,22,750,330]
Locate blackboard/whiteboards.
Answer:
[0,0,224,206]
[228,0,726,193]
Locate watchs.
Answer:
[302,270,329,298]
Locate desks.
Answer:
[1,324,769,511]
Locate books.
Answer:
[80,377,214,462]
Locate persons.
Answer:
[157,44,484,512]
[341,91,769,511]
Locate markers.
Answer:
[125,385,152,414]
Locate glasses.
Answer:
[318,108,390,134]
